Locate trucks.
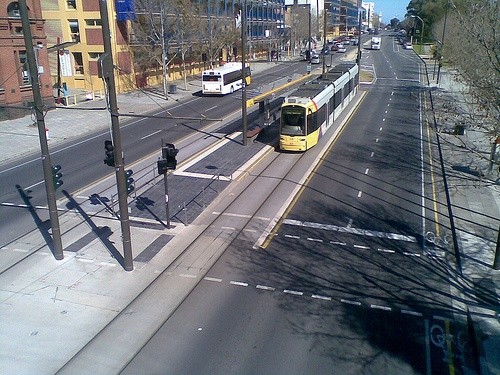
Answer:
[371,36,382,49]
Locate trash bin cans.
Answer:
[455,120,466,135]
[170,85,176,94]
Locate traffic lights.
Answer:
[103,140,114,167]
[52,164,64,188]
[124,169,134,194]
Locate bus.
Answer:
[279,62,360,152]
[201,62,251,96]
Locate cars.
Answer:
[311,55,320,64]
[396,33,413,50]
[321,36,358,54]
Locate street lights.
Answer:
[411,15,424,54]
